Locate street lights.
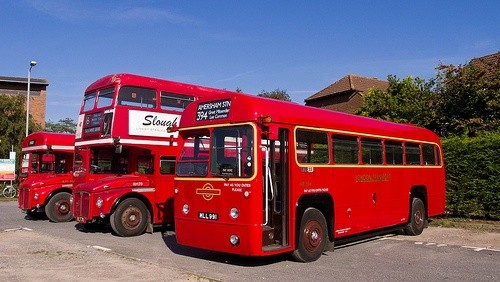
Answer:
[26,61,37,138]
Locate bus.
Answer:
[18,131,76,222]
[173,98,446,264]
[70,73,259,237]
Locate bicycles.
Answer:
[1,185,17,199]
